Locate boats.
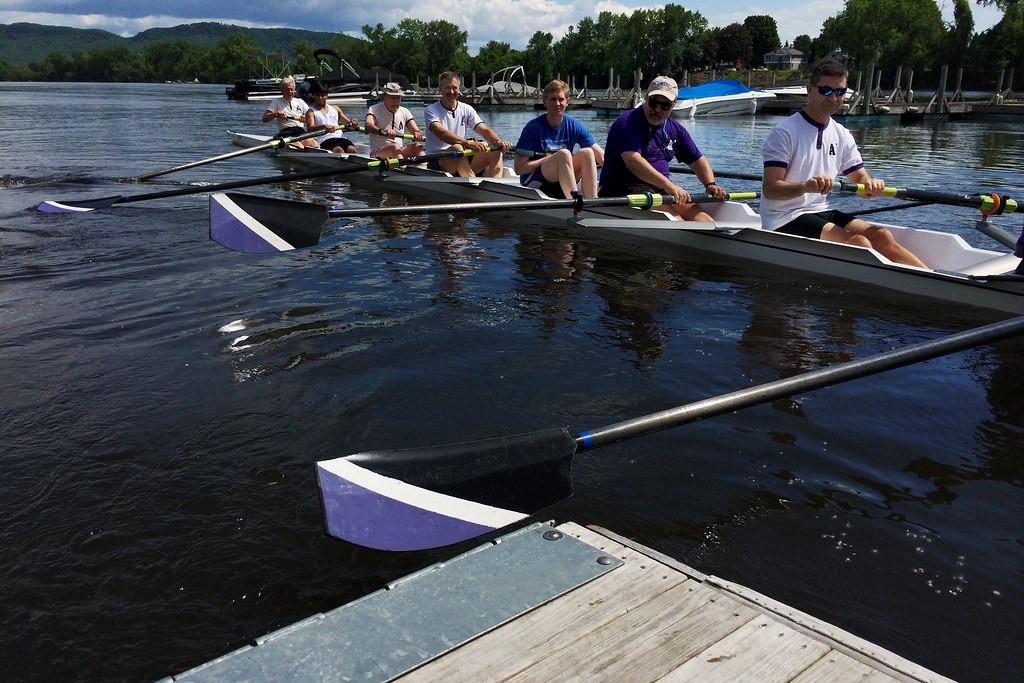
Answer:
[760,81,858,108]
[226,54,318,101]
[226,130,1024,316]
[294,49,417,106]
[633,79,778,117]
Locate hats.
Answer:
[646,75,678,101]
[382,82,405,96]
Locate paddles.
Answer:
[273,110,367,135]
[132,120,354,183]
[379,125,428,141]
[314,315,1017,553]
[829,176,1023,218]
[207,189,763,258]
[513,147,764,183]
[34,144,505,215]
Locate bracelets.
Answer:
[379,128,384,135]
[705,182,718,187]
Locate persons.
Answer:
[424,71,513,178]
[514,79,605,200]
[760,58,930,270]
[365,82,428,169]
[262,78,318,149]
[306,82,359,154]
[598,76,726,222]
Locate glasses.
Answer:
[649,96,672,111]
[814,84,847,96]
